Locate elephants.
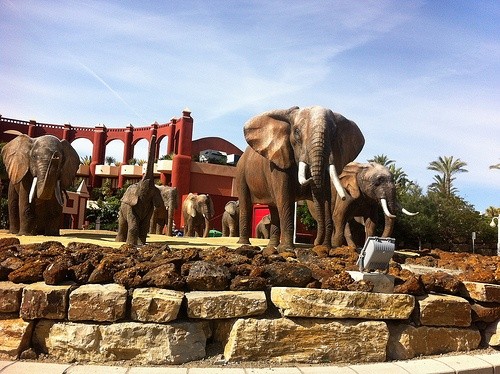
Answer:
[306,161,420,251]
[255,214,271,239]
[182,192,213,237]
[222,200,240,237]
[233,106,365,253]
[1,135,80,236]
[116,135,155,244]
[149,185,178,236]
